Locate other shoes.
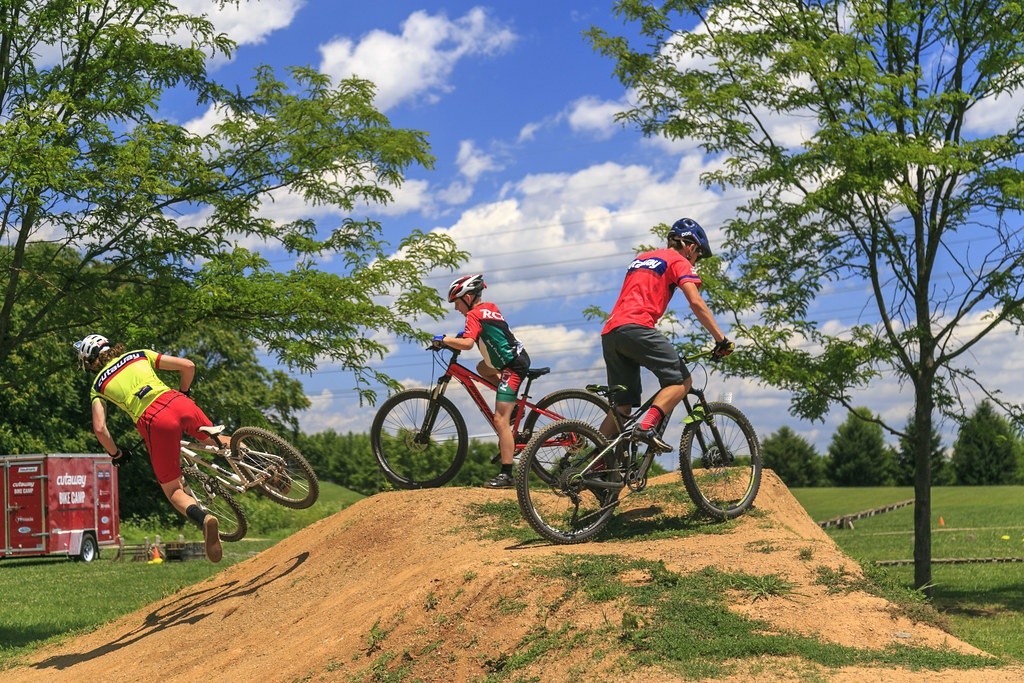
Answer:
[203,514,222,563]
[267,469,290,493]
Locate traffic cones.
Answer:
[939,516,945,526]
[153,546,161,559]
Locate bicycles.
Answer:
[369,336,627,485]
[113,398,320,542]
[516,339,764,546]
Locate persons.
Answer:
[432,274,531,489]
[78,334,248,563]
[586,218,733,508]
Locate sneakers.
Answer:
[630,423,673,452]
[489,474,515,488]
[510,404,525,425]
[585,470,620,504]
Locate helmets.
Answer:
[448,274,487,302]
[668,217,711,258]
[78,334,110,371]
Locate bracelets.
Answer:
[108,449,120,458]
